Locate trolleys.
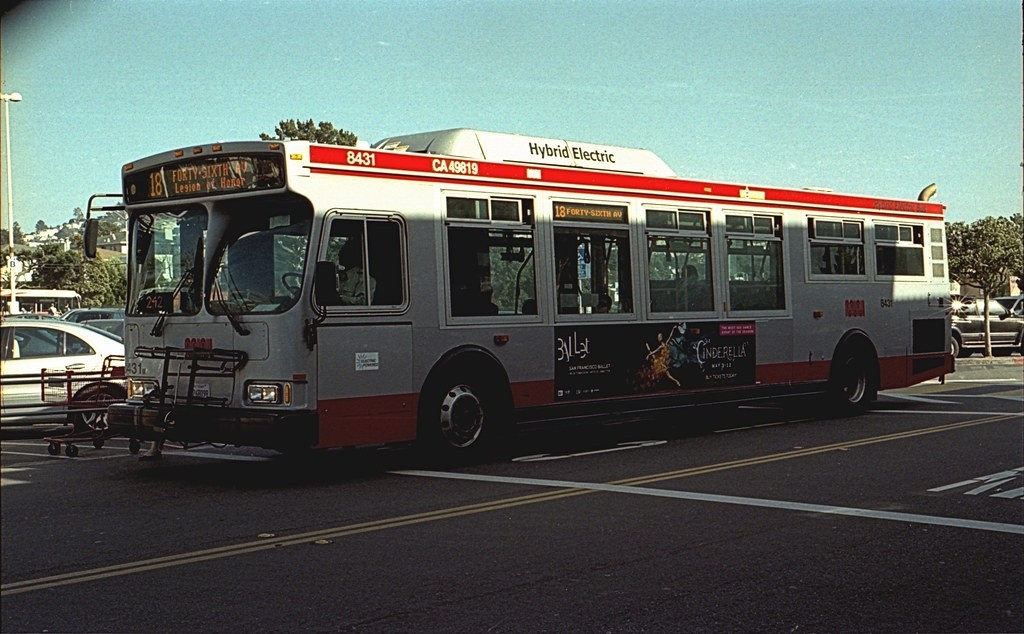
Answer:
[41,355,141,458]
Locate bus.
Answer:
[0,289,82,318]
[83,126,956,471]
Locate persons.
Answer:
[676,265,713,311]
[47,303,57,315]
[21,308,27,314]
[339,238,376,305]
[30,307,34,313]
[63,304,69,314]
[139,441,165,458]
[595,295,612,313]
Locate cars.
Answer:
[1,321,125,424]
[951,295,1024,358]
[993,295,1024,316]
[0,307,126,338]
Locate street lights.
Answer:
[0,93,23,314]
